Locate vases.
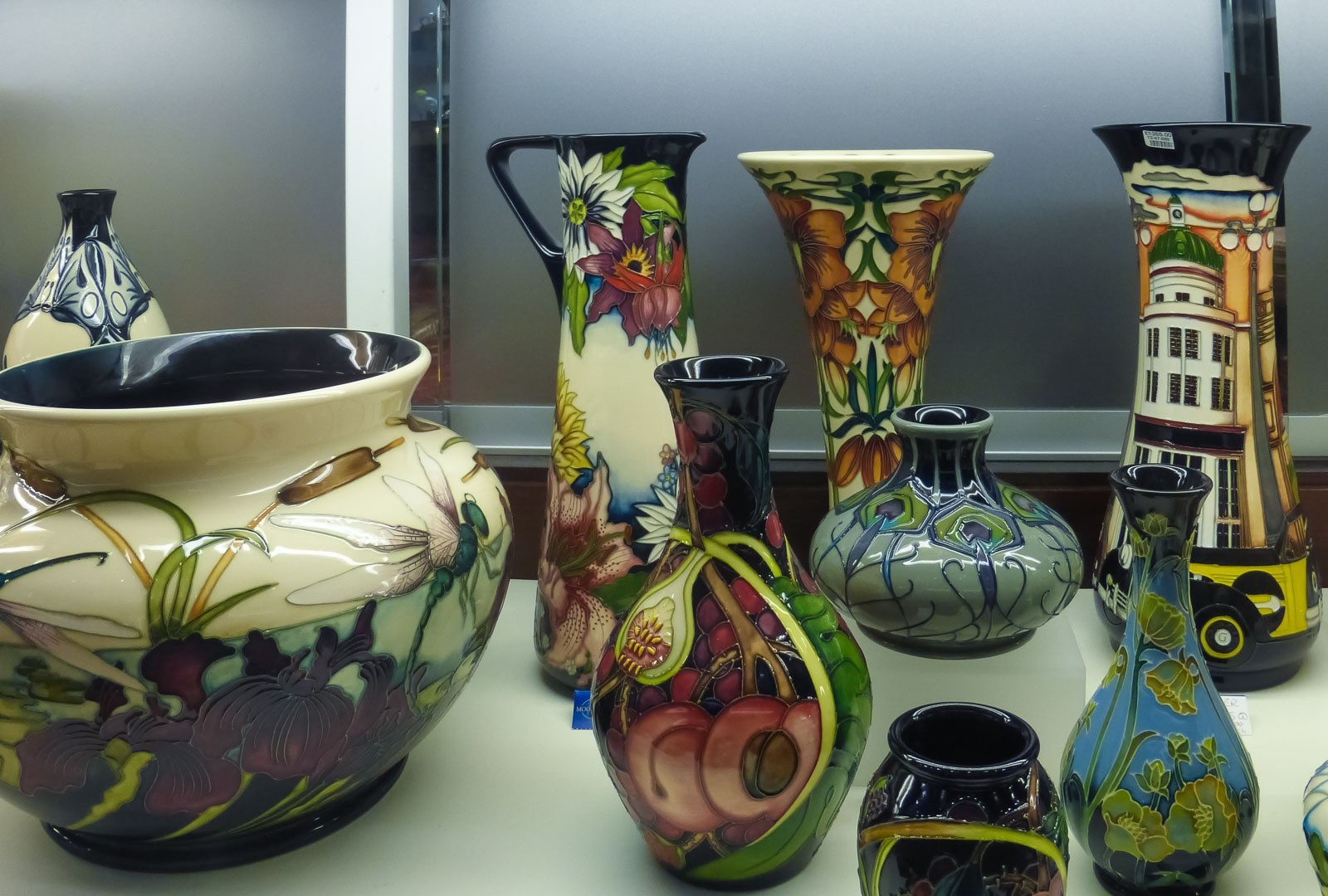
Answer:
[809,402,1087,659]
[1061,463,1260,896]
[1094,122,1323,693]
[490,130,708,689]
[588,358,874,892]
[856,701,1070,896]
[0,327,516,873]
[3,190,170,371]
[738,149,994,508]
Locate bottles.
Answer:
[0,117,1328,896]
